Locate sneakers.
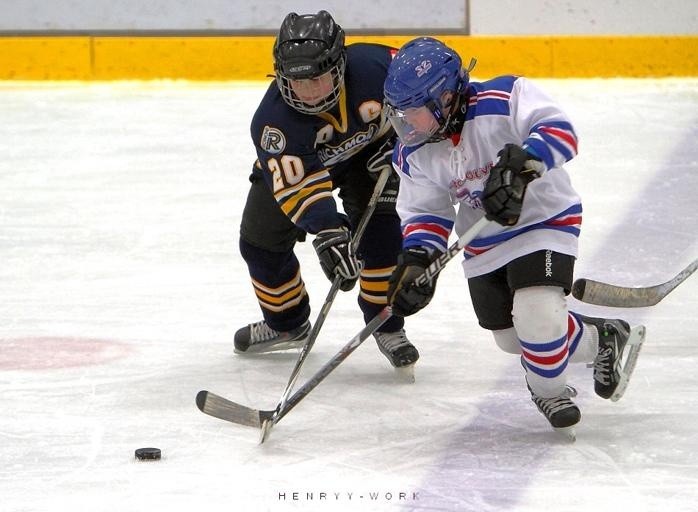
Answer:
[524,374,581,428]
[233,320,312,353]
[593,317,631,399]
[372,328,419,367]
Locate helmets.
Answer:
[272,10,348,116]
[382,37,462,148]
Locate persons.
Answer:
[233,10,420,368]
[383,37,631,430]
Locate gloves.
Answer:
[312,225,366,291]
[387,247,436,317]
[480,144,548,227]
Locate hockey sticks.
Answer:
[259,167,391,445]
[571,259,698,308]
[195,214,494,428]
